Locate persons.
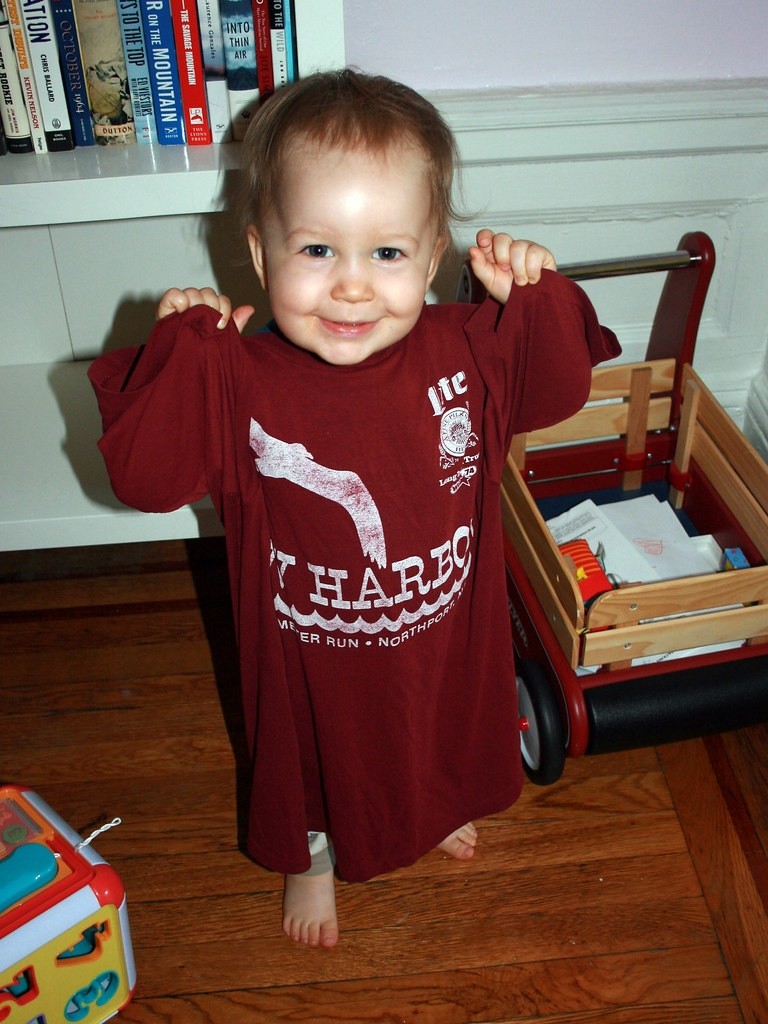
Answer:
[89,70,620,947]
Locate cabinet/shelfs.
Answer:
[0,0,346,552]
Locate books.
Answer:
[0,1,296,158]
